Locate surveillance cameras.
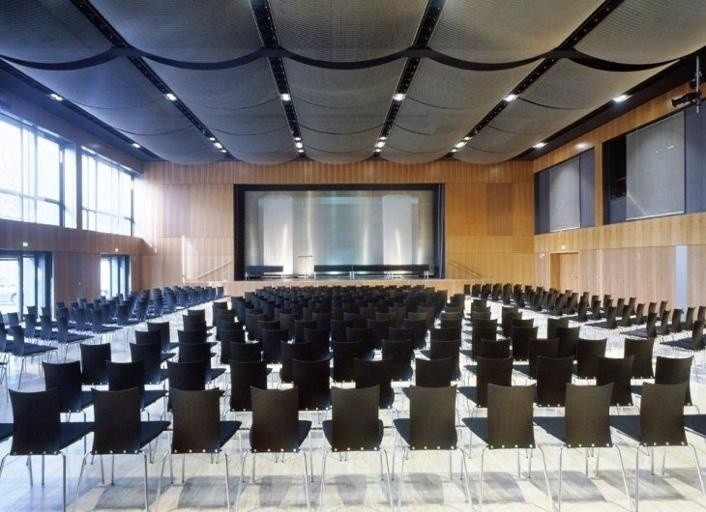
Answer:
[670,92,701,107]
